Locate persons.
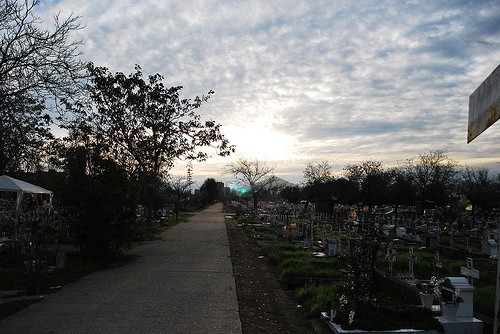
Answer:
[27,193,50,222]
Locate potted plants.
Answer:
[438,279,461,318]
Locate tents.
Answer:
[0,175,54,224]
